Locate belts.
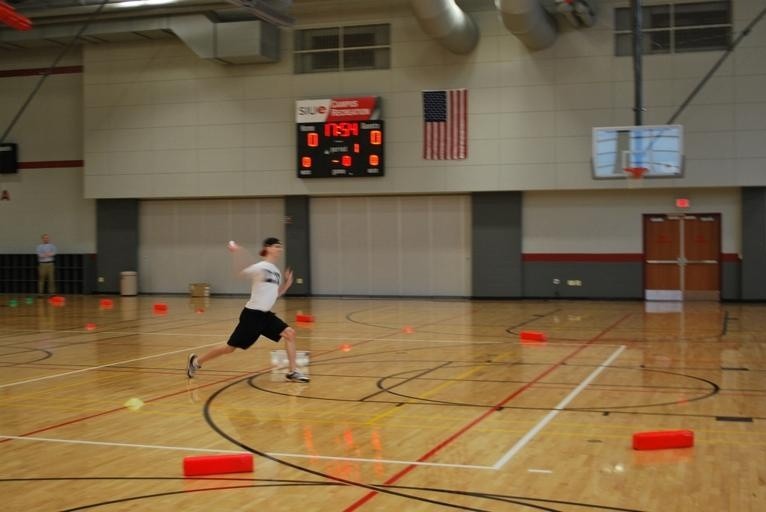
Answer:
[39,261,54,263]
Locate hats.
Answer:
[258,237,281,256]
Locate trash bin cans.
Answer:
[120,271,138,296]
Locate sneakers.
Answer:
[284,369,310,383]
[186,352,202,379]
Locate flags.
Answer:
[420,89,468,160]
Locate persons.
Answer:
[186,237,307,382]
[35,232,58,296]
